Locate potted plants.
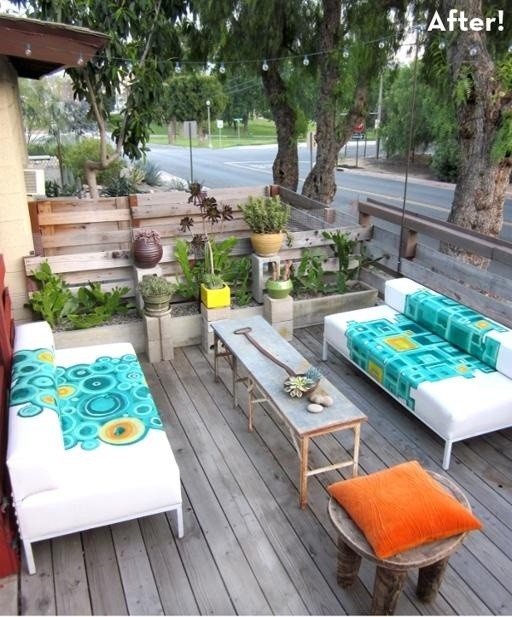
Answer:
[200,275,231,308]
[133,230,163,268]
[236,194,290,256]
[265,260,293,298]
[137,274,178,316]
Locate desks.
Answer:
[210,315,367,508]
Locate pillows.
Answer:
[326,461,483,560]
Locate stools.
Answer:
[329,471,473,616]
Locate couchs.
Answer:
[6,320,186,575]
[322,277,512,471]
